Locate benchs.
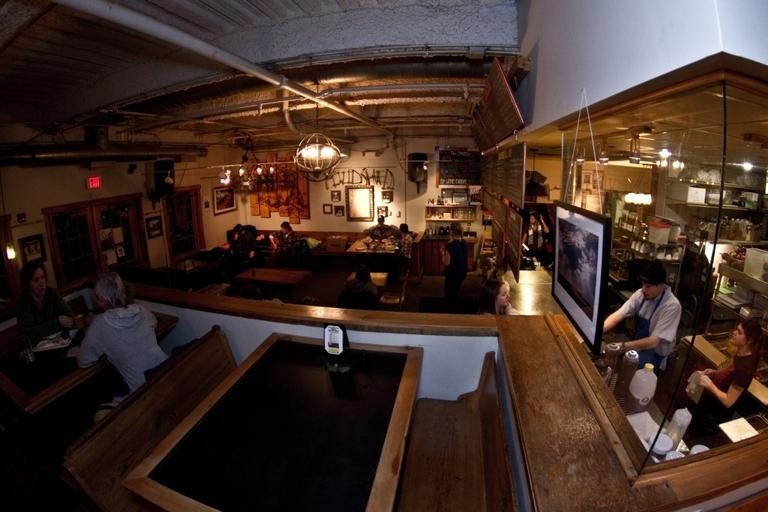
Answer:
[614,288,634,317]
[65,324,238,512]
[1,288,97,364]
[397,352,526,512]
[398,232,425,287]
[379,269,408,311]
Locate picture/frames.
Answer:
[382,191,394,203]
[18,233,47,266]
[451,192,468,204]
[323,204,332,214]
[334,206,344,217]
[331,191,341,202]
[440,187,470,206]
[551,199,612,356]
[212,186,237,216]
[146,216,163,239]
[377,206,388,218]
[345,185,374,222]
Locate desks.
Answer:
[122,332,425,512]
[234,267,312,300]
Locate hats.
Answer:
[639,261,666,283]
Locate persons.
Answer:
[347,264,378,295]
[522,211,549,258]
[281,221,295,252]
[444,223,467,298]
[602,258,683,377]
[397,223,412,268]
[698,319,763,430]
[77,271,169,406]
[369,215,392,239]
[477,279,519,316]
[15,260,73,337]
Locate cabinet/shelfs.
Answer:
[656,162,768,248]
[419,233,477,275]
[609,208,686,337]
[704,261,768,386]
[423,203,482,236]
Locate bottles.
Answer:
[623,363,657,412]
[615,350,639,395]
[604,342,619,368]
[666,408,692,451]
[617,212,649,241]
[598,359,612,385]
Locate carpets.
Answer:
[417,295,480,315]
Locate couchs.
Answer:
[214,230,415,278]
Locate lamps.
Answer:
[208,135,292,193]
[3,239,16,264]
[294,84,342,182]
[598,133,610,163]
[627,125,655,163]
